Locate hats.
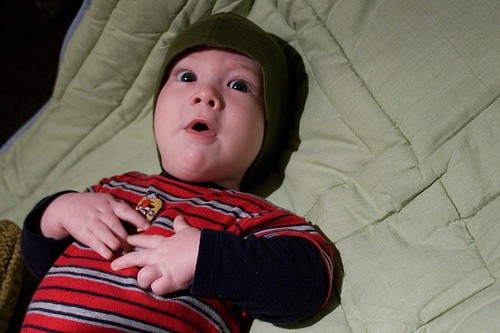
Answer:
[153,12,289,191]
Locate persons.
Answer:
[2,11,337,333]
